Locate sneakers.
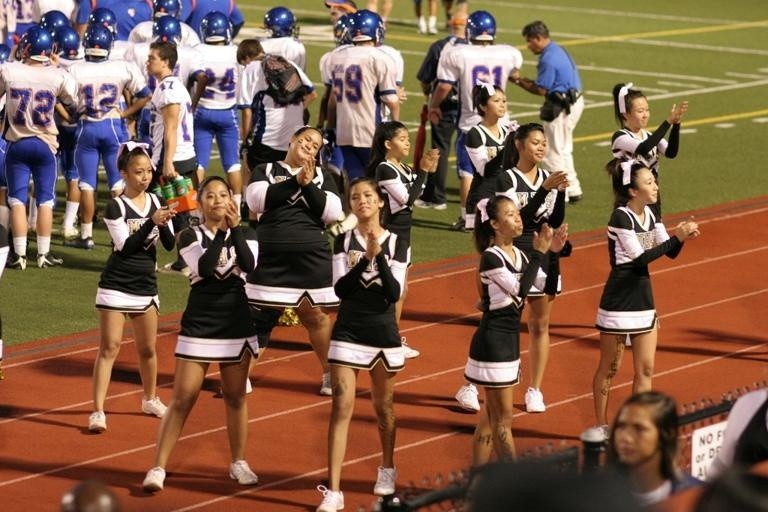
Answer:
[320,371,335,396]
[524,386,546,413]
[87,410,108,432]
[77,237,94,251]
[452,216,475,233]
[142,467,166,490]
[315,484,346,512]
[228,459,259,486]
[581,424,611,444]
[401,336,420,360]
[163,262,189,276]
[453,383,481,413]
[569,192,583,202]
[5,253,28,271]
[372,465,399,496]
[413,199,448,211]
[141,396,168,419]
[246,377,253,395]
[418,22,427,34]
[63,235,80,246]
[429,21,438,34]
[35,251,66,268]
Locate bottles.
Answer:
[175,175,188,196]
[184,176,194,191]
[164,181,176,199]
[151,182,163,198]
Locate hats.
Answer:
[323,0,357,13]
[447,11,469,25]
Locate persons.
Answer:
[316,181,404,512]
[317,14,403,184]
[465,196,567,467]
[515,18,584,203]
[593,157,700,431]
[697,389,767,510]
[135,41,200,276]
[197,11,242,217]
[370,122,439,360]
[129,0,198,59]
[78,8,149,80]
[415,10,484,210]
[328,10,400,181]
[72,1,154,42]
[584,392,707,510]
[88,140,177,431]
[454,123,572,413]
[442,0,468,30]
[427,11,521,230]
[178,0,243,42]
[238,38,319,156]
[0,26,77,270]
[140,175,260,490]
[464,78,516,179]
[66,23,152,248]
[605,83,689,225]
[245,59,310,174]
[230,127,340,395]
[253,7,305,74]
[365,0,392,31]
[413,0,440,33]
[123,16,192,96]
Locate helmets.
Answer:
[82,22,112,62]
[17,26,53,64]
[152,0,181,18]
[88,7,118,41]
[333,12,351,47]
[38,10,69,33]
[465,10,497,42]
[198,11,234,45]
[347,9,385,47]
[151,14,183,46]
[51,26,80,61]
[263,5,294,38]
[0,44,11,63]
